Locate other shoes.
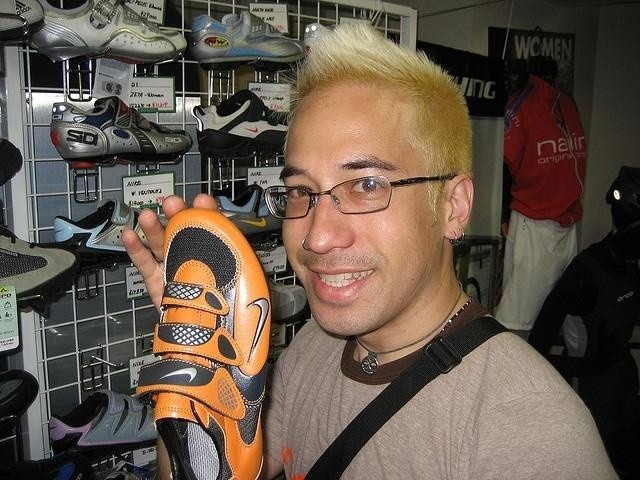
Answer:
[1,138,22,185]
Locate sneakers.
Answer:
[0,368,39,433]
[54,199,168,272]
[0,227,81,305]
[31,0,188,65]
[135,205,273,480]
[48,390,161,459]
[1,1,44,42]
[268,282,307,322]
[191,89,295,157]
[189,9,307,65]
[0,449,95,480]
[50,96,193,169]
[213,184,287,240]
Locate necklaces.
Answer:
[355,281,466,376]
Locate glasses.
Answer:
[265,174,457,221]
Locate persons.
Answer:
[121,20,617,479]
[496,54,586,331]
